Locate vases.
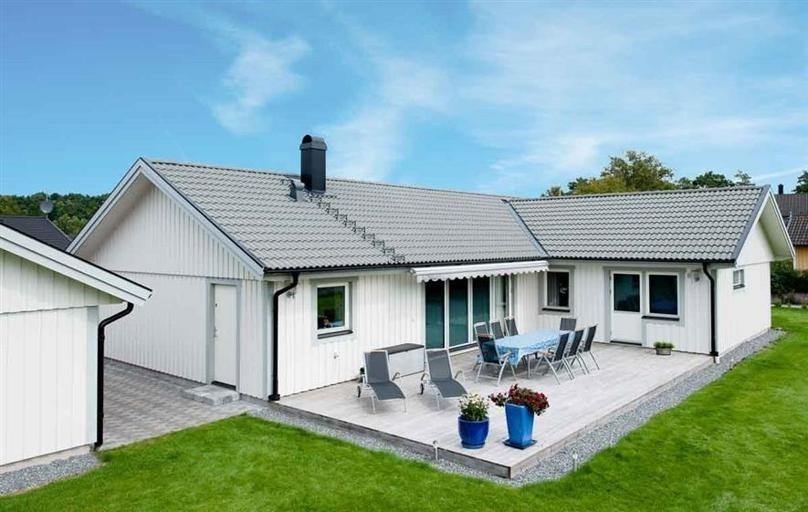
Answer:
[457,417,490,449]
[502,402,537,451]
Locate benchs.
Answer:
[377,343,425,379]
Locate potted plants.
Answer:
[653,342,674,355]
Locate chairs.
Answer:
[419,347,468,411]
[356,349,407,414]
[472,315,600,385]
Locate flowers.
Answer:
[487,383,549,416]
[456,393,491,420]
